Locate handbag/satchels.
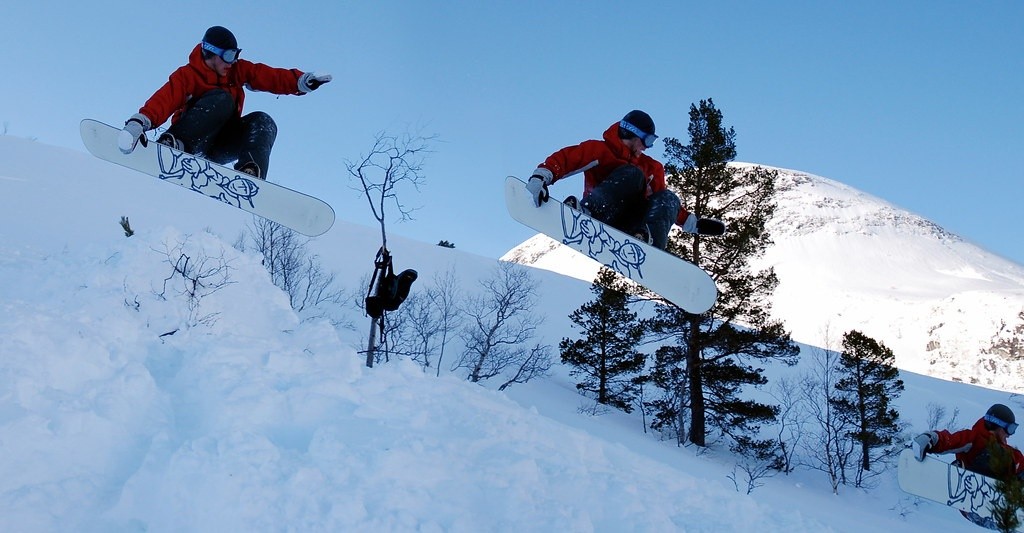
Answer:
[365,268,418,319]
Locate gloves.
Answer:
[525,168,554,207]
[297,71,331,93]
[683,214,725,235]
[911,431,938,461]
[118,113,152,154]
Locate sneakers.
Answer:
[563,196,592,216]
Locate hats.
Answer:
[202,26,237,59]
[619,110,655,140]
[984,404,1016,430]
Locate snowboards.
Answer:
[79,118,336,238]
[897,448,1024,533]
[503,175,718,315]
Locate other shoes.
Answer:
[239,162,261,178]
[156,133,179,150]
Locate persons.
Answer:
[526,110,725,251]
[912,403,1024,529]
[118,26,332,180]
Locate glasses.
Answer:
[221,48,242,64]
[1005,423,1019,435]
[643,134,658,148]
